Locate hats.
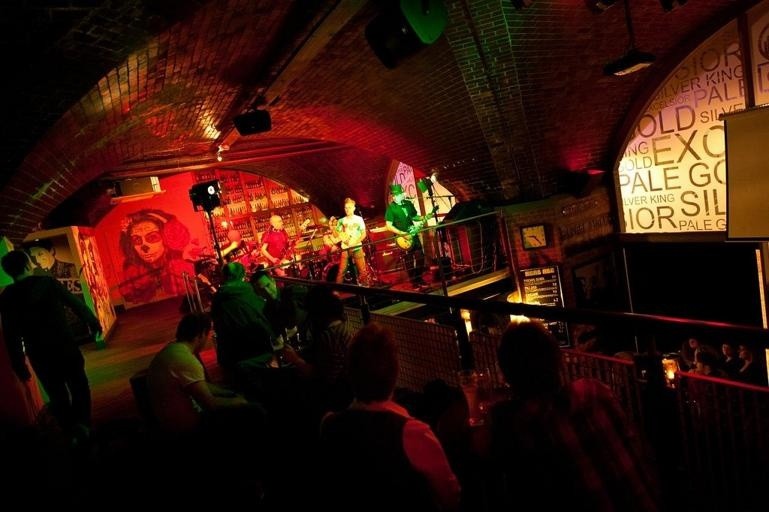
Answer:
[387,184,403,195]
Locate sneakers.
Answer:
[411,284,421,290]
[418,280,428,287]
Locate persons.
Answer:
[383,184,430,289]
[677,335,758,415]
[213,228,254,263]
[331,197,368,297]
[145,259,659,511]
[260,215,291,275]
[117,209,196,303]
[0,248,105,460]
[321,217,339,234]
[22,240,80,288]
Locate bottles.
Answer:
[196,173,315,250]
[493,359,512,401]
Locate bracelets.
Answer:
[423,216,426,220]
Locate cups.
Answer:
[284,325,307,358]
[267,333,290,367]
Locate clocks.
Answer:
[520,223,551,250]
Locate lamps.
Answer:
[234,96,272,136]
[366,0,449,69]
[593,0,686,76]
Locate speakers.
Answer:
[117,176,160,196]
[235,108,272,136]
[189,181,223,208]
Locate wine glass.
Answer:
[458,368,494,428]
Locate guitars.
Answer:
[268,218,311,276]
[198,272,220,295]
[395,206,439,250]
